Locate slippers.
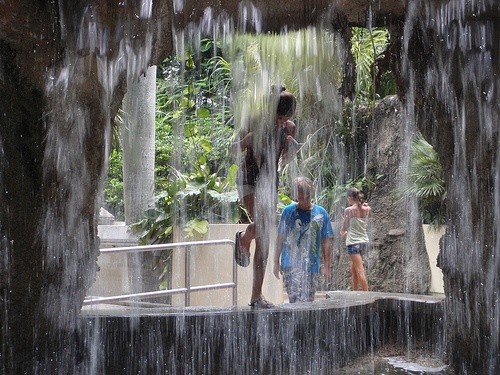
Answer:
[236,231,250,267]
[248,297,275,308]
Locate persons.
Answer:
[273,176,333,303]
[234,84,296,309]
[339,187,372,290]
